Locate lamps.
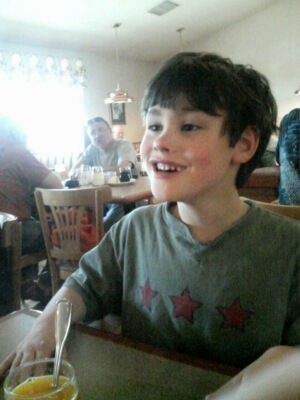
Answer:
[105,23,134,104]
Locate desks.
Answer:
[62,176,154,215]
[0,310,243,400]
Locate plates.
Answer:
[105,178,136,186]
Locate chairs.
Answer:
[34,185,113,327]
[0,222,47,318]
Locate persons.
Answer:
[270,108,300,205]
[68,117,139,229]
[0,51,300,400]
[0,119,63,303]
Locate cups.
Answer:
[82,165,93,184]
[92,167,104,186]
[4,358,80,400]
[119,167,131,182]
